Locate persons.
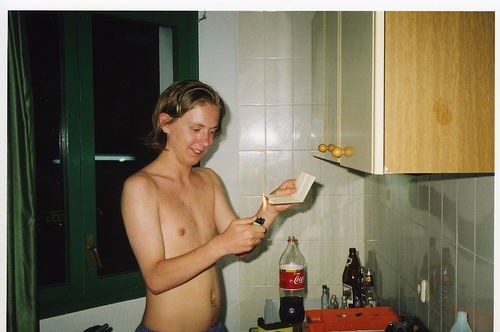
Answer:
[120,80,303,332]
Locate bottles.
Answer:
[329,299,335,309]
[450,312,472,332]
[321,285,329,309]
[360,267,376,308]
[332,295,338,309]
[279,237,305,323]
[343,248,360,308]
[340,296,347,309]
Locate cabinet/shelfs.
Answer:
[309,11,495,175]
